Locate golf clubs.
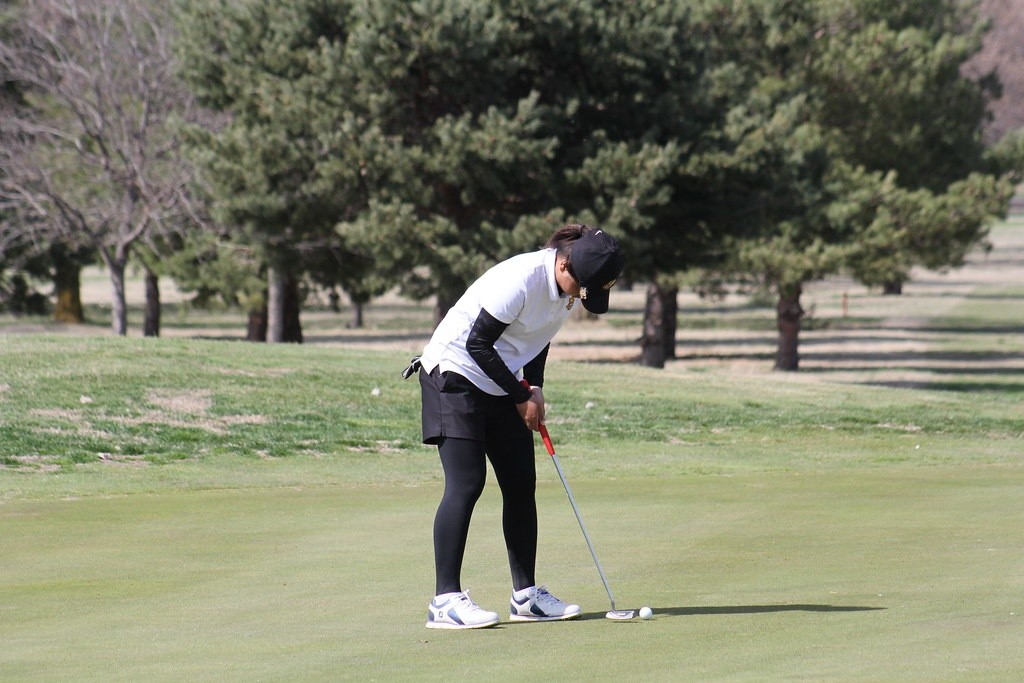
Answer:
[518,379,635,620]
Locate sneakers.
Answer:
[509,587,583,623]
[425,590,499,629]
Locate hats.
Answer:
[566,228,624,314]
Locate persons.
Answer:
[418,220,626,630]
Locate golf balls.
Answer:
[638,606,653,620]
